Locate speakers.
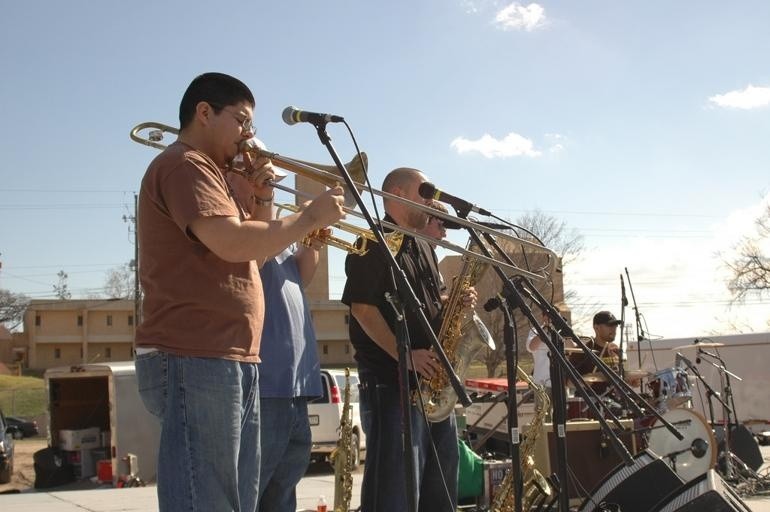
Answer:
[578,449,686,512]
[654,469,753,512]
[717,425,764,485]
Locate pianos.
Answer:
[462,378,537,395]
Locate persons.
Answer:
[340,167,478,511]
[525,304,576,423]
[225,134,321,511]
[565,310,641,419]
[136,72,345,512]
[416,201,456,249]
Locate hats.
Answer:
[593,311,624,325]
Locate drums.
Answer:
[633,407,717,482]
[648,366,692,407]
[566,397,615,421]
[618,393,652,419]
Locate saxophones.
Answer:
[487,364,552,512]
[329,368,354,512]
[409,217,497,423]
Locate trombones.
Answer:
[130,123,559,282]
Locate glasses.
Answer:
[204,100,257,137]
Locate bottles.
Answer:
[317,496,327,512]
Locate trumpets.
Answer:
[273,203,405,258]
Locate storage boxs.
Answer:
[58,427,111,484]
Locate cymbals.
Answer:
[579,371,650,382]
[563,348,599,353]
[674,343,724,349]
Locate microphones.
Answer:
[282,105,343,125]
[483,276,524,312]
[694,339,703,362]
[639,335,648,341]
[443,217,508,230]
[419,183,491,218]
[620,275,629,306]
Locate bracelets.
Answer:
[254,191,276,207]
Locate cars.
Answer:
[0,410,38,484]
[307,369,367,472]
